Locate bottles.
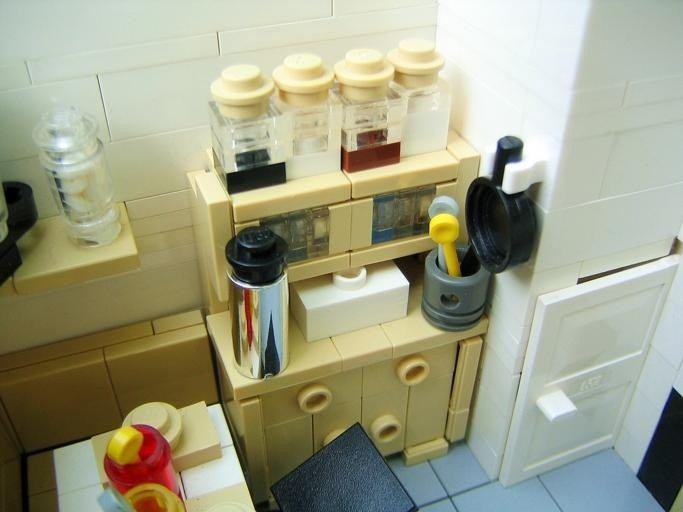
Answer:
[31,95,122,250]
[102,425,186,512]
[259,183,434,264]
[222,224,292,384]
